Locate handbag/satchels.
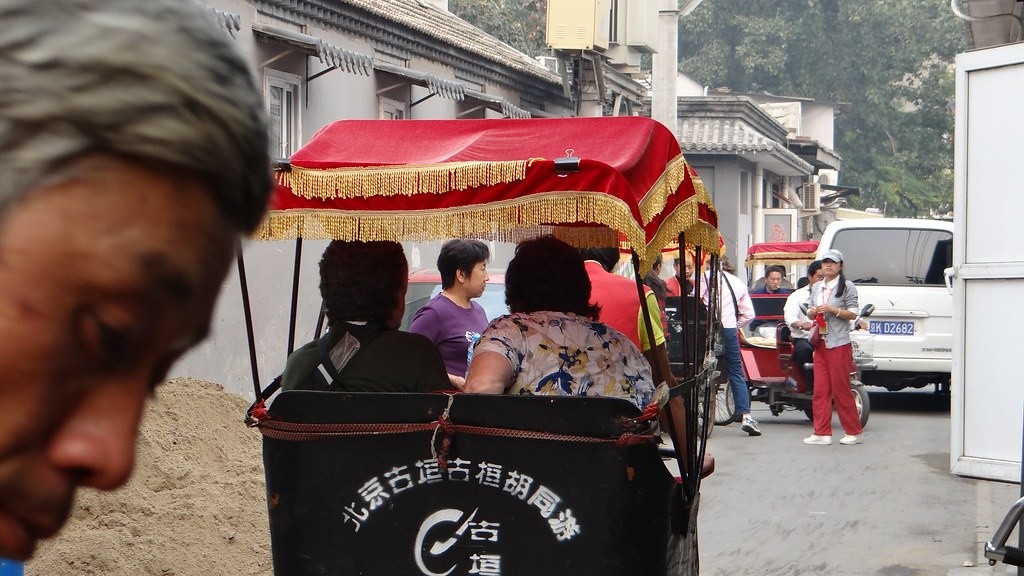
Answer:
[807,323,821,345]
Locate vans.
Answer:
[814,218,956,392]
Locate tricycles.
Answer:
[747,242,820,290]
[724,290,875,430]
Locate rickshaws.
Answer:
[236,115,739,576]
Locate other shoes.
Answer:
[805,383,814,395]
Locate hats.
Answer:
[821,249,845,263]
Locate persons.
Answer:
[282,236,454,393]
[0,0,278,576]
[635,248,867,445]
[571,243,715,479]
[410,238,490,392]
[463,234,662,439]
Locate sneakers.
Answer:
[803,434,832,445]
[840,435,864,445]
[741,416,762,435]
